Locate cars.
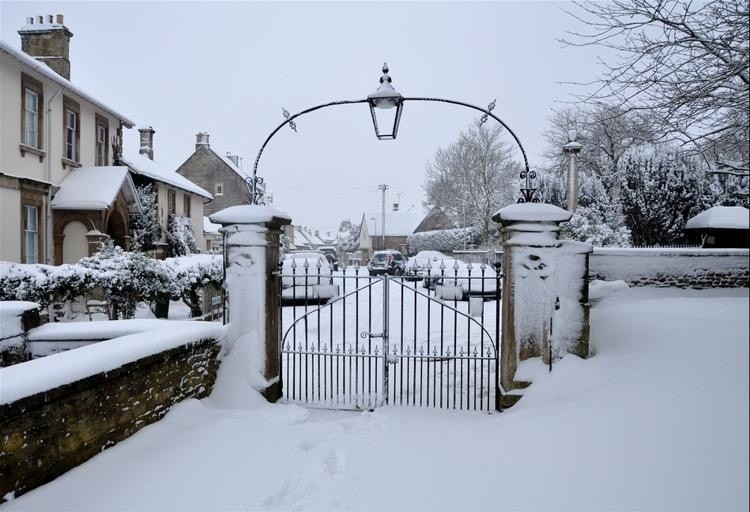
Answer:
[405,250,502,300]
[280,246,339,304]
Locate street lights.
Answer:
[561,128,584,210]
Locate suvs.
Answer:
[368,249,407,278]
